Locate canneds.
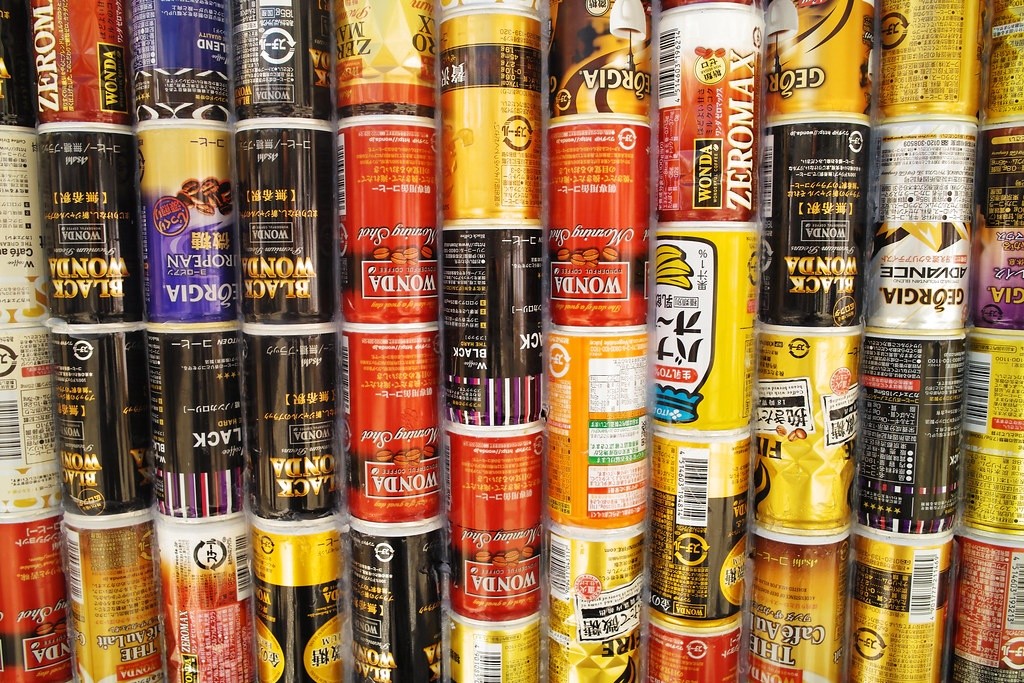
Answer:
[0,0,1024,683]
